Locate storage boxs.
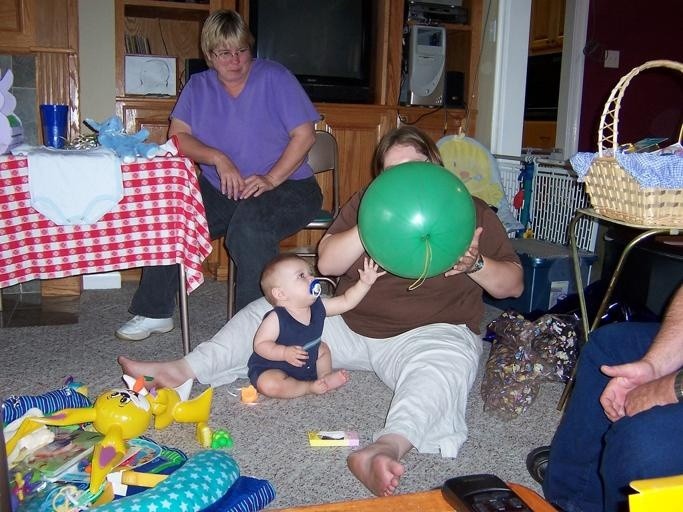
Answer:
[483,238,597,318]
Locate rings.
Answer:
[253,184,260,191]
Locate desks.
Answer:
[1,150,214,356]
[556,207,683,412]
[253,481,560,512]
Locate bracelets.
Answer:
[674,371,683,402]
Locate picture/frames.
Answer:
[122,54,179,98]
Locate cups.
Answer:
[39,104,70,147]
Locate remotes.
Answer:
[441,474,532,512]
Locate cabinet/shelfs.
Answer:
[523,120,557,153]
[398,10,472,111]
[528,2,567,57]
[1,1,83,299]
[223,105,381,280]
[114,1,219,283]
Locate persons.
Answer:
[247,253,387,399]
[115,11,324,342]
[526,282,683,512]
[118,127,525,495]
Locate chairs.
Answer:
[227,130,340,319]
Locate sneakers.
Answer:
[116,313,174,340]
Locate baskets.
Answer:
[575,60,683,228]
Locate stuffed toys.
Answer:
[84,114,159,163]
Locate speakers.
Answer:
[445,69,465,108]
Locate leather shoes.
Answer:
[526,445,553,485]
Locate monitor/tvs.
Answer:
[249,0,373,102]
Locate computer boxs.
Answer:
[406,26,446,108]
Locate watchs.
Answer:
[466,253,484,273]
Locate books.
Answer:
[123,35,154,55]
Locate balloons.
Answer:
[357,162,477,291]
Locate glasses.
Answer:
[210,48,249,62]
